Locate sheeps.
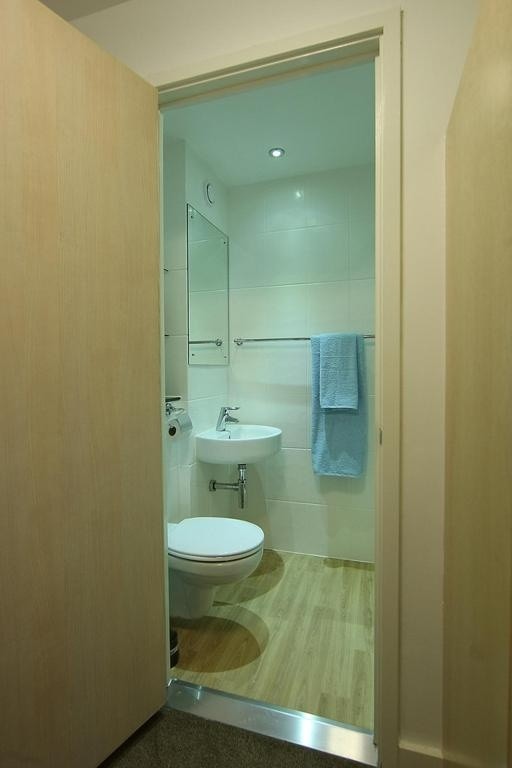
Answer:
[217,404,240,431]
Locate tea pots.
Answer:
[187,203,230,367]
